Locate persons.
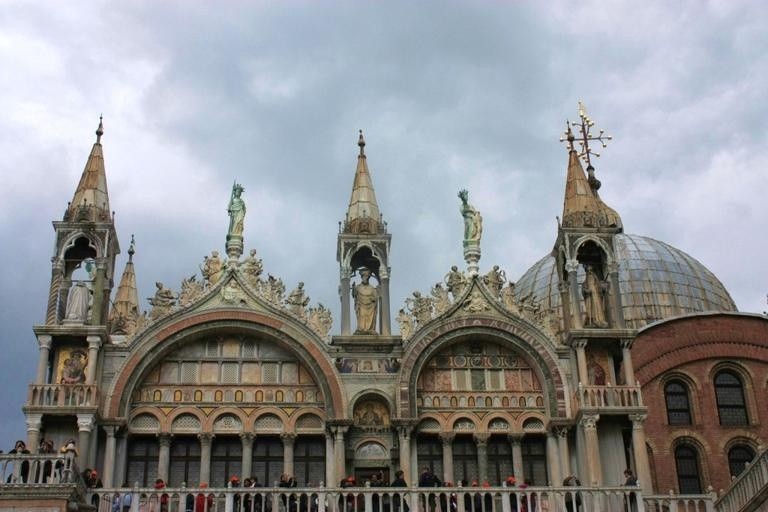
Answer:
[621,469,637,511]
[118,248,331,332]
[587,356,609,405]
[352,268,379,334]
[562,475,582,511]
[228,185,246,236]
[458,189,482,240]
[8,441,78,483]
[81,466,539,512]
[53,357,86,405]
[62,280,90,321]
[356,404,384,429]
[581,265,609,328]
[397,265,557,339]
